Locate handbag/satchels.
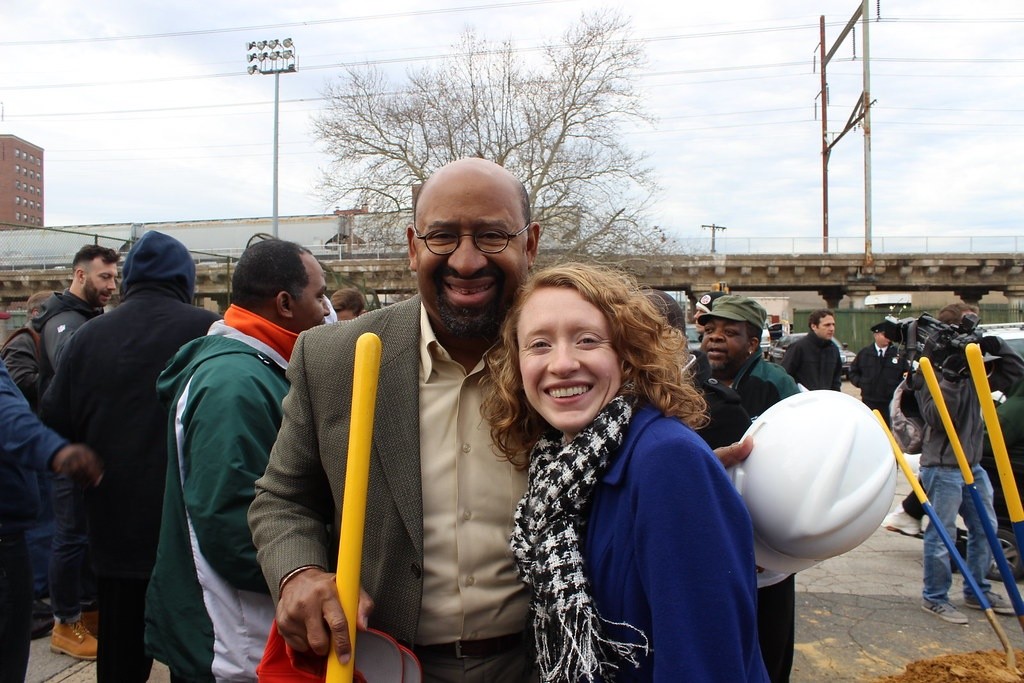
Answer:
[193,549,282,683]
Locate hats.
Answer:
[257,607,422,683]
[695,291,726,312]
[699,295,767,335]
[870,321,886,332]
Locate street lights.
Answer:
[246,37,298,238]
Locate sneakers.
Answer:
[881,504,921,535]
[965,592,1015,614]
[921,597,969,624]
[50,613,100,660]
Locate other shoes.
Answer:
[30,618,55,639]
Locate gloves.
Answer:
[942,355,967,382]
[980,336,1000,356]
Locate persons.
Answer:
[329,289,367,322]
[32,244,120,375]
[848,324,909,429]
[479,263,769,683]
[694,292,801,683]
[781,309,842,392]
[0,357,101,683]
[249,158,753,683]
[914,302,1024,624]
[42,230,223,683]
[641,288,752,451]
[0,290,57,408]
[143,239,331,683]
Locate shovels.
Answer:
[873,409,1024,677]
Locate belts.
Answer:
[415,630,527,661]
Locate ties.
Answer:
[879,350,883,362]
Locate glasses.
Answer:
[411,221,535,256]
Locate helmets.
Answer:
[726,386,897,573]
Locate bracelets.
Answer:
[279,565,324,598]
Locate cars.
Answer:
[768,332,857,381]
[684,321,794,360]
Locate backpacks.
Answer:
[887,378,928,453]
